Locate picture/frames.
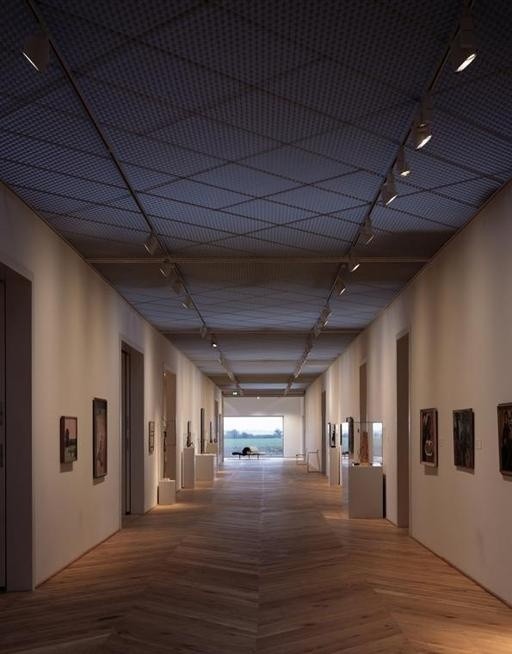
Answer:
[419,402,512,477]
[60,398,108,478]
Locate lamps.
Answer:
[346,39,479,276]
[14,27,53,75]
[143,234,193,310]
